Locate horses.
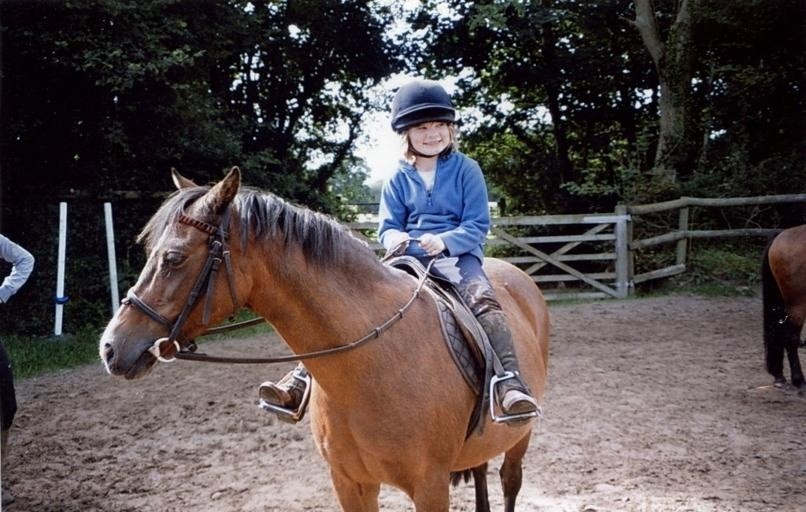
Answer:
[98,165,551,512]
[761,224,806,396]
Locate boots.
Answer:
[475,310,539,428]
[257,360,313,425]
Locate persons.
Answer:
[257,79,539,428]
[0,232,35,307]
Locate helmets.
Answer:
[389,77,459,132]
